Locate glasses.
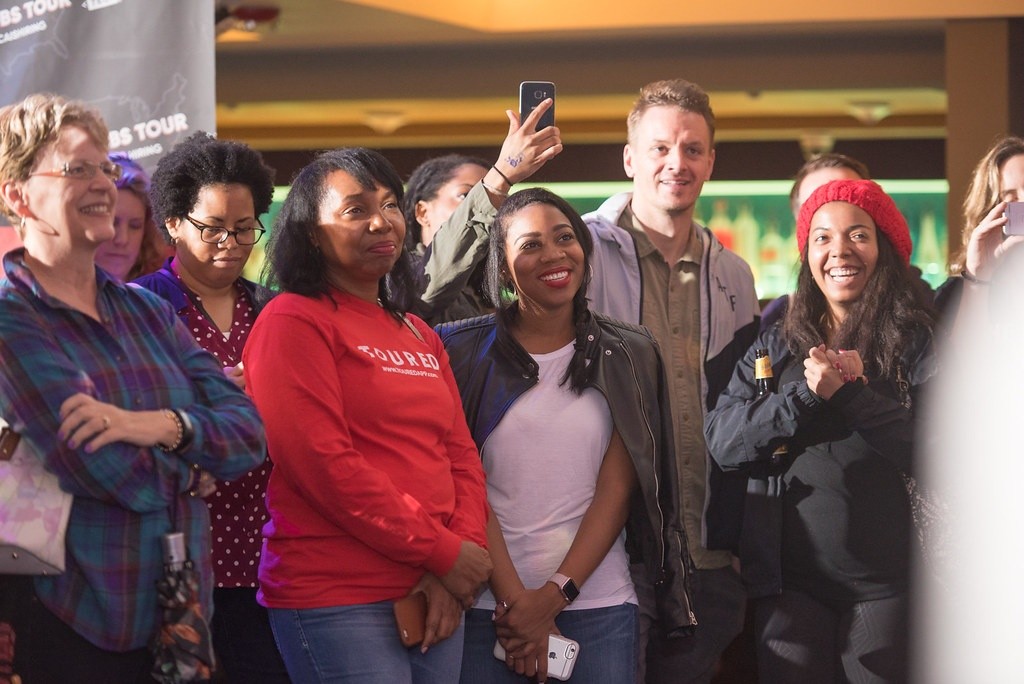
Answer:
[26,161,119,180]
[182,213,268,246]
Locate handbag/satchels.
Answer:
[0,414,74,576]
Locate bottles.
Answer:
[755,347,788,455]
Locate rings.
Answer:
[101,415,111,429]
[500,601,510,610]
[509,655,514,659]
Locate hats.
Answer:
[797,178,913,268]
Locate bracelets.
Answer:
[161,409,194,453]
[961,270,992,285]
[492,164,513,187]
[156,408,182,452]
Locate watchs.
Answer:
[547,572,580,605]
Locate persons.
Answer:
[433,188,698,684]
[577,82,762,684]
[404,98,563,330]
[0,79,280,684]
[243,148,496,684]
[703,136,1024,684]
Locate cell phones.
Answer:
[519,81,557,132]
[493,633,580,682]
[1002,202,1024,236]
[393,591,428,647]
